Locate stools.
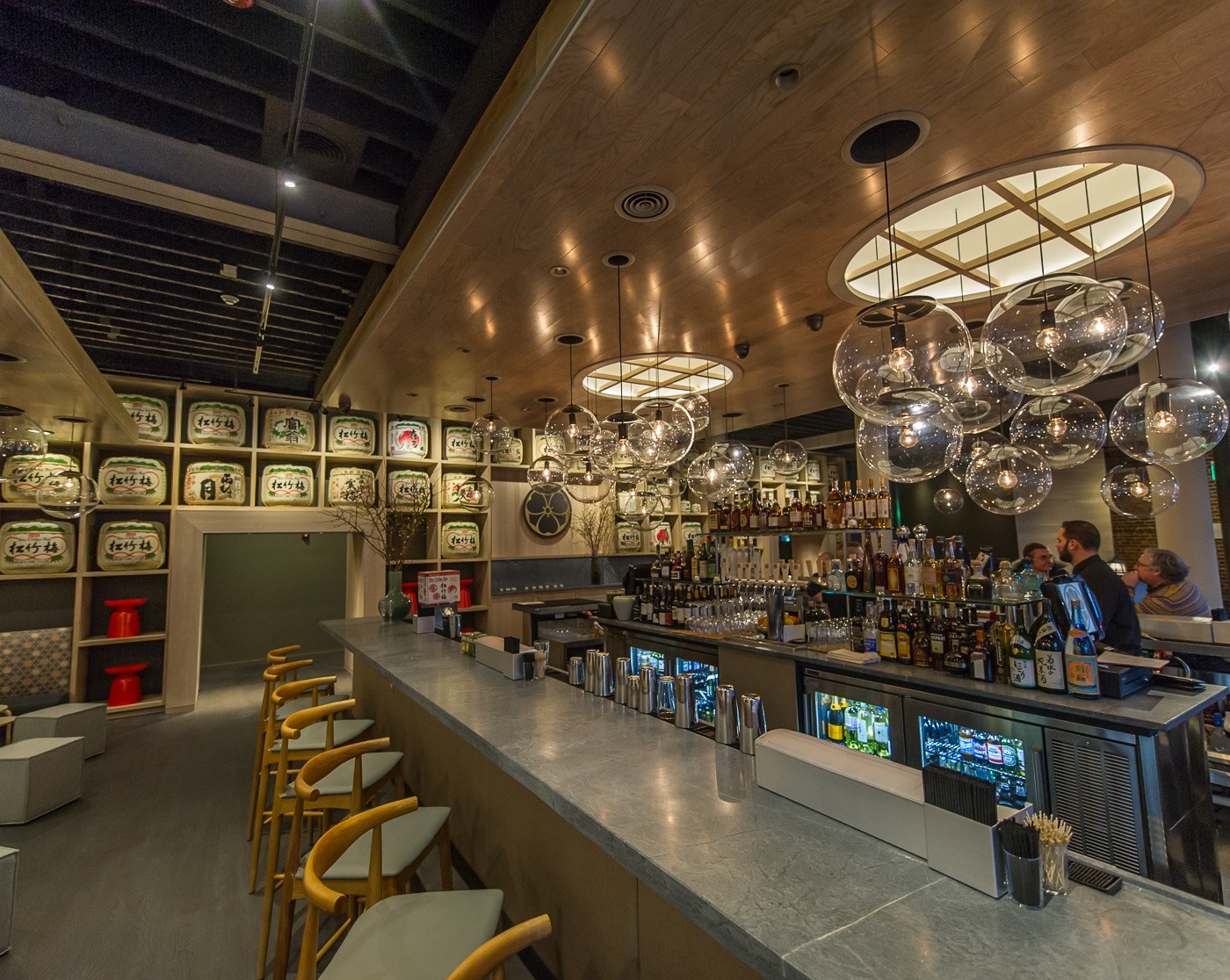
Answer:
[255,697,407,980]
[245,658,352,844]
[448,911,554,980]
[247,674,377,897]
[296,793,506,980]
[8,690,68,716]
[0,737,86,825]
[272,736,454,979]
[11,702,107,760]
[0,846,21,956]
[265,644,332,775]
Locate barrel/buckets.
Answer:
[443,614,462,638]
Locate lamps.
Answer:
[459,131,1230,533]
[0,408,48,479]
[35,421,101,518]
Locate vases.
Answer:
[565,483,622,584]
[312,466,450,619]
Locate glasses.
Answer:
[1027,554,1053,559]
[1137,560,1149,568]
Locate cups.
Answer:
[739,692,767,755]
[521,661,546,681]
[1039,843,1068,895]
[534,639,549,669]
[627,674,637,710]
[1001,842,1044,911]
[673,672,699,729]
[615,657,630,704]
[568,648,613,697]
[714,685,739,744]
[639,665,655,714]
[656,675,676,720]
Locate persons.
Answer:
[1054,520,1144,658]
[1009,541,1070,579]
[1117,549,1212,619]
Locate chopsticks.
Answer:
[1002,811,1041,908]
[522,654,535,680]
[921,763,998,827]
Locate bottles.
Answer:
[633,474,1100,704]
[638,651,719,725]
[920,717,1026,809]
[1204,694,1230,754]
[828,696,890,759]
